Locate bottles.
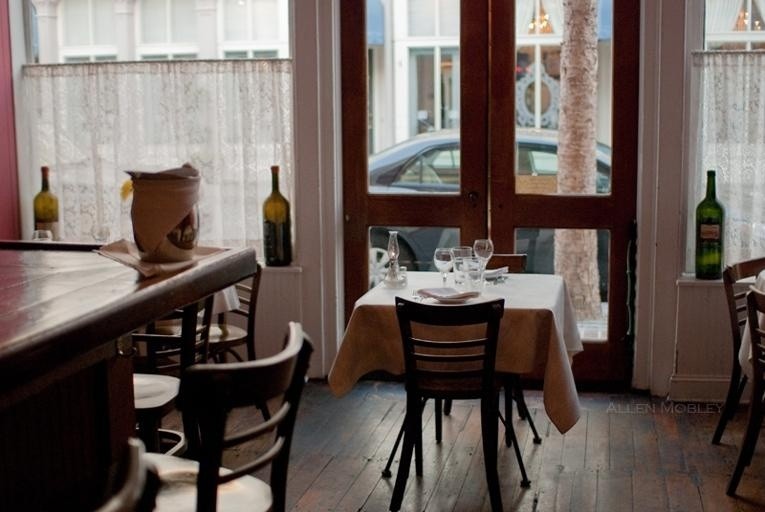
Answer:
[32,165,62,242]
[695,168,724,280]
[262,165,294,268]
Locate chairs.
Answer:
[132,301,201,455]
[441,253,530,418]
[726,286,765,498]
[389,296,506,512]
[142,321,314,511]
[709,257,765,448]
[142,259,276,433]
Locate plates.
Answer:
[430,295,472,304]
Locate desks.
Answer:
[328,270,584,488]
[736,269,764,473]
[1,239,255,511]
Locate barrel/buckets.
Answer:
[131,178,200,263]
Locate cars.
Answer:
[365,124,612,305]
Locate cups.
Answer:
[433,239,494,296]
